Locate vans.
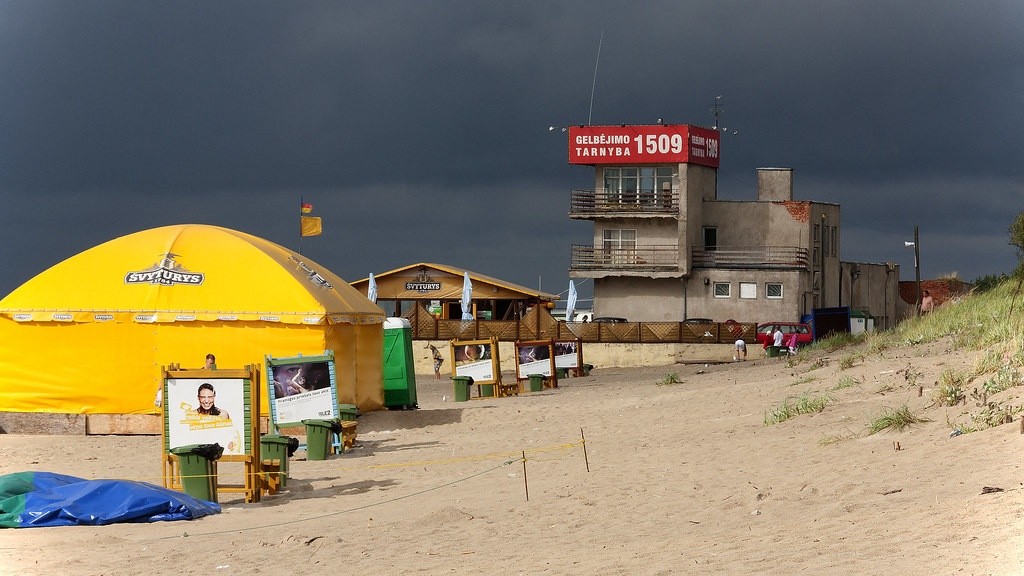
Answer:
[755,321,813,346]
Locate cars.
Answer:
[683,318,713,324]
[588,316,628,323]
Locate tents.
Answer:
[0,223,387,416]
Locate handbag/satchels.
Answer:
[786,339,790,346]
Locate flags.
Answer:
[300,203,322,236]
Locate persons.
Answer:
[582,315,588,323]
[201,354,216,370]
[518,343,577,364]
[763,326,800,356]
[272,364,311,399]
[430,345,444,382]
[920,290,934,316]
[189,383,229,420]
[734,336,747,362]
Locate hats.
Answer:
[766,331,771,335]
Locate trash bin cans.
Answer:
[450,375,474,402]
[582,363,591,377]
[169,443,224,505]
[481,384,494,397]
[339,403,359,421]
[555,368,566,379]
[259,434,293,487]
[303,419,334,461]
[527,373,547,392]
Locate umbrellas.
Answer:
[462,272,474,320]
[565,279,577,322]
[367,272,378,303]
